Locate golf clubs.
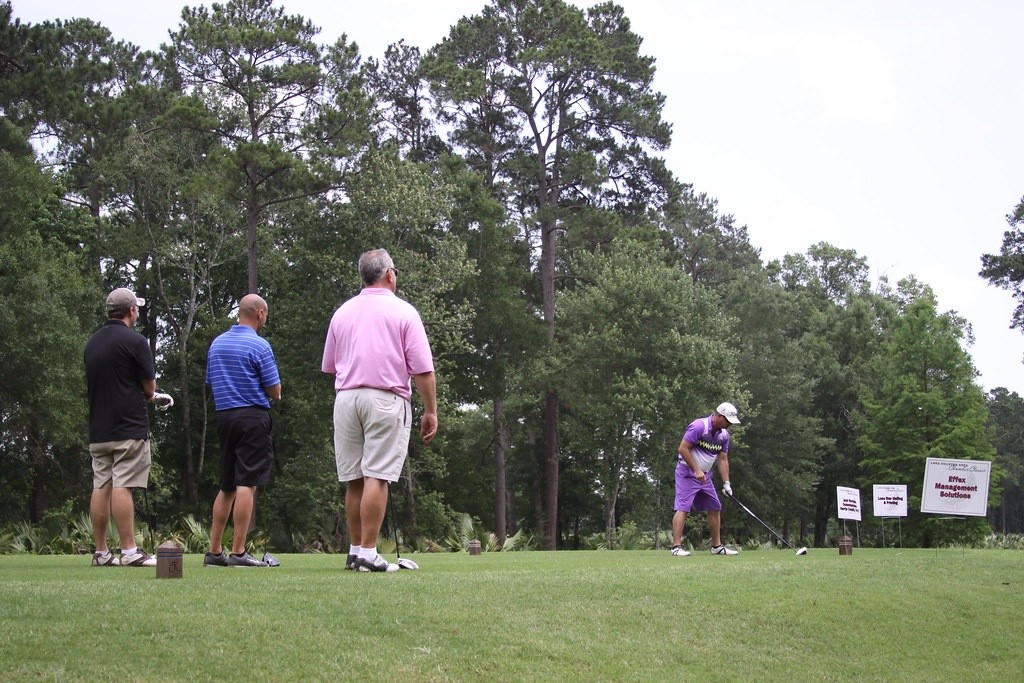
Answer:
[388,483,421,570]
[143,487,159,558]
[262,482,280,566]
[722,486,808,556]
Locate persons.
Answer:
[670,401,741,556]
[320,249,438,572]
[84,288,174,568]
[204,294,282,568]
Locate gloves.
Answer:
[722,481,733,498]
[152,392,174,411]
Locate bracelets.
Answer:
[722,481,730,484]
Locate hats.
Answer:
[717,402,741,424]
[108,288,145,307]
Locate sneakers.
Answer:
[671,547,690,557]
[227,552,270,568]
[203,551,229,568]
[711,545,737,556]
[344,554,358,570]
[120,550,157,566]
[92,552,121,566]
[355,556,402,573]
